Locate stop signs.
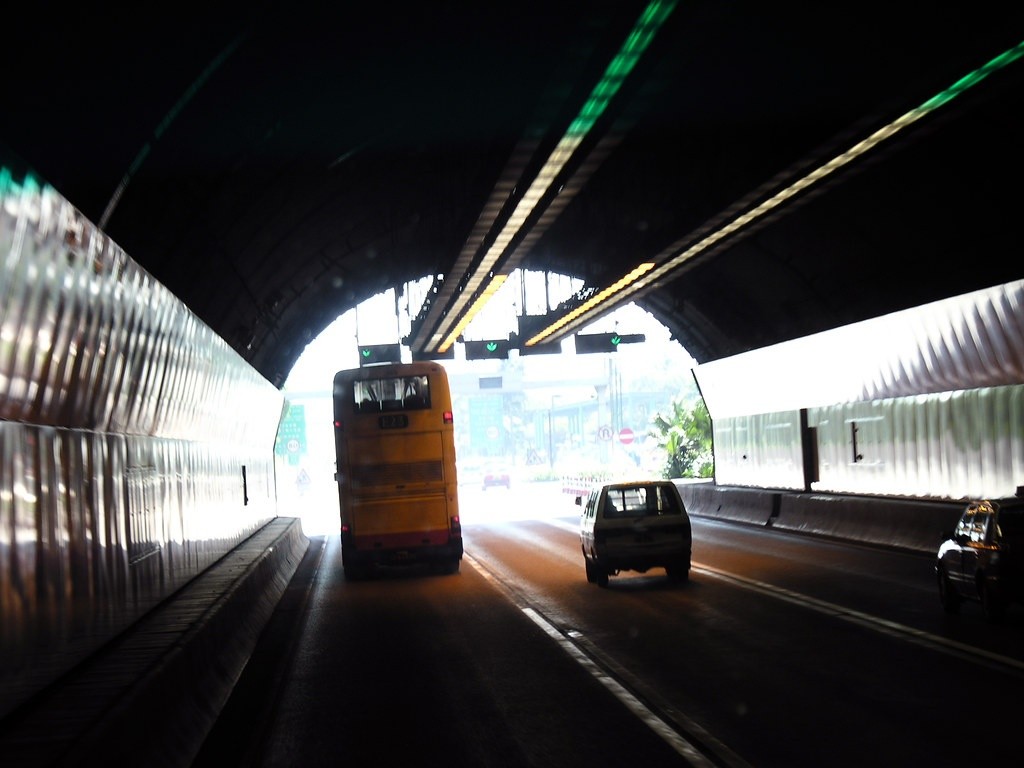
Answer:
[618,426,635,445]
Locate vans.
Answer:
[575,480,693,588]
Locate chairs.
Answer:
[354,403,360,411]
[360,401,381,413]
[404,395,423,410]
[381,400,402,411]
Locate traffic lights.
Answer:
[465,338,510,361]
[573,332,620,355]
[358,344,402,365]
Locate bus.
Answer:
[332,362,464,581]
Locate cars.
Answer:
[481,468,511,490]
